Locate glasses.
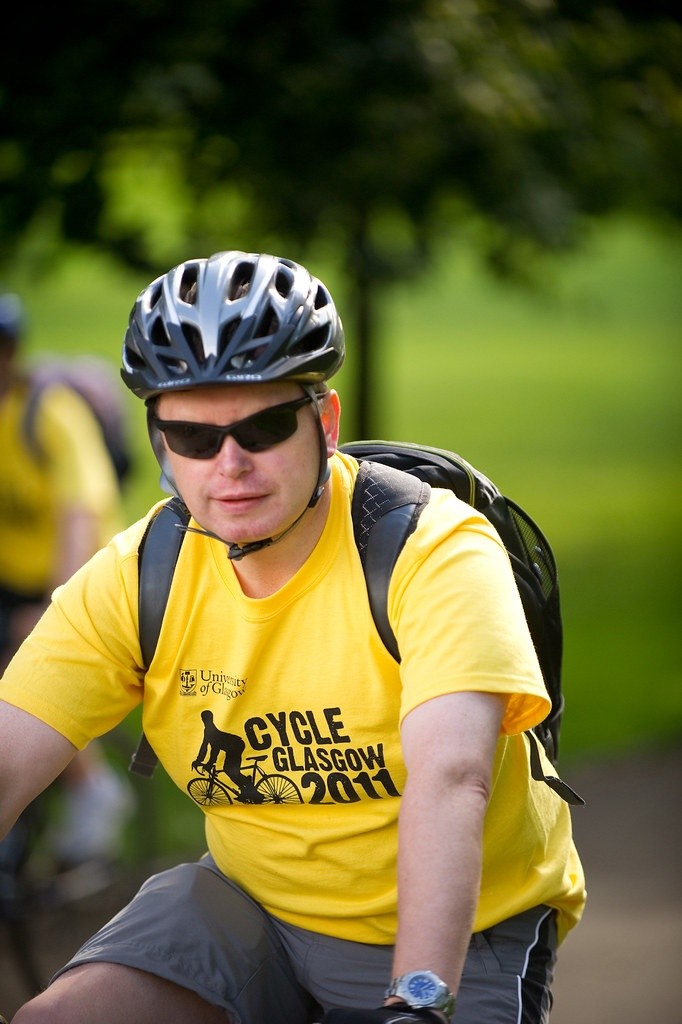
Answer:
[148,391,328,460]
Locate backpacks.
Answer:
[23,362,132,486]
[126,440,586,807]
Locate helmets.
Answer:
[120,251,346,400]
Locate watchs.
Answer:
[384,969,456,1018]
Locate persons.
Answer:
[0,249,586,1024]
[0,296,136,871]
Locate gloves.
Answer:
[323,1002,445,1024]
